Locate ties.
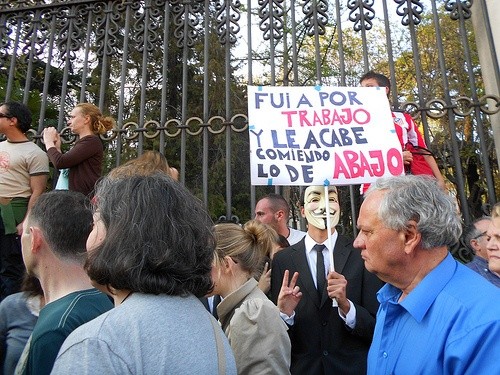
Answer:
[314,244,326,301]
[213,294,221,320]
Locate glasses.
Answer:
[0,112,12,119]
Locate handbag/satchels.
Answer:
[405,142,436,178]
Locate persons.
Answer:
[352,175,500,375]
[268,185,383,375]
[359,74,444,184]
[0,102,49,302]
[50,173,236,375]
[19,189,113,375]
[464,204,500,292]
[207,196,307,375]
[42,102,113,196]
[0,275,47,375]
